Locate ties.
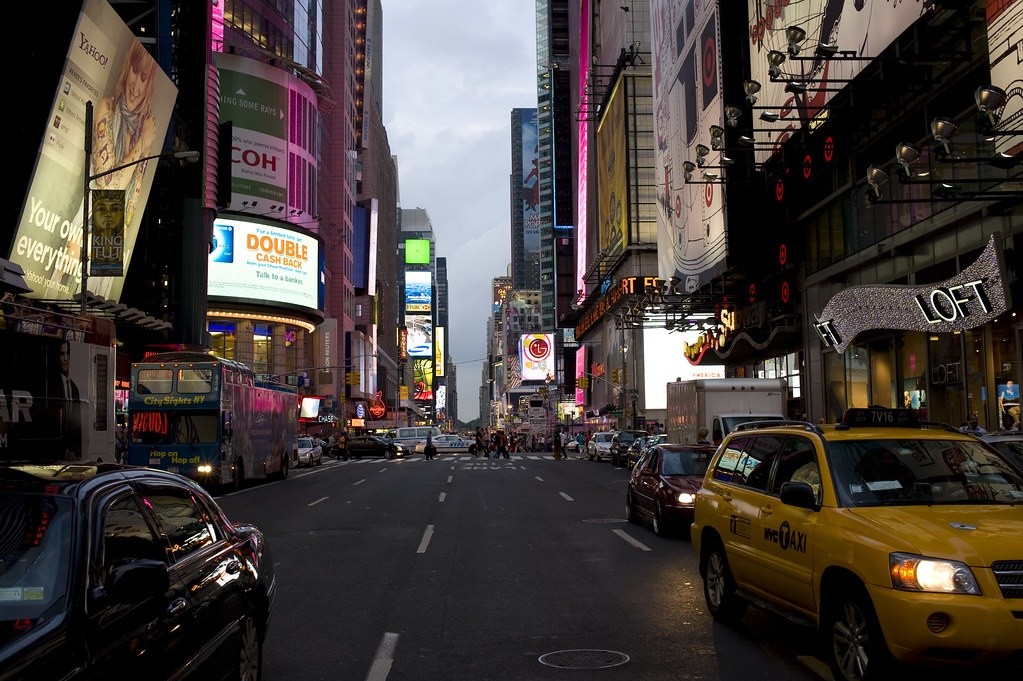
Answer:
[66,380,71,417]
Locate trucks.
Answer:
[665,377,788,448]
[382,427,441,454]
[0,256,117,466]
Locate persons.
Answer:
[299,431,350,462]
[114,430,129,465]
[1000,415,1019,432]
[794,406,807,422]
[712,430,723,447]
[960,413,987,434]
[42,340,83,462]
[389,434,397,460]
[475,423,665,460]
[695,427,710,445]
[425,430,434,460]
[41,40,169,300]
[1001,382,1021,414]
[903,390,912,410]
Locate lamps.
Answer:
[864,162,1023,204]
[570,249,721,330]
[573,56,616,122]
[26,290,173,332]
[930,116,1023,165]
[216,200,347,245]
[894,141,1023,186]
[682,27,882,186]
[972,84,1023,136]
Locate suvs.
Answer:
[690,415,1023,681]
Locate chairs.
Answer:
[771,449,852,506]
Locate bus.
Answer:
[123,350,299,488]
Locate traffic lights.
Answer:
[575,376,588,389]
[341,394,345,404]
[612,368,621,383]
[346,372,360,385]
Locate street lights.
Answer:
[580,370,628,427]
[340,353,380,429]
[77,146,207,320]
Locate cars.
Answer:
[0,463,278,681]
[945,433,1023,503]
[588,430,668,469]
[297,437,323,468]
[331,435,411,459]
[416,434,477,456]
[624,442,720,537]
